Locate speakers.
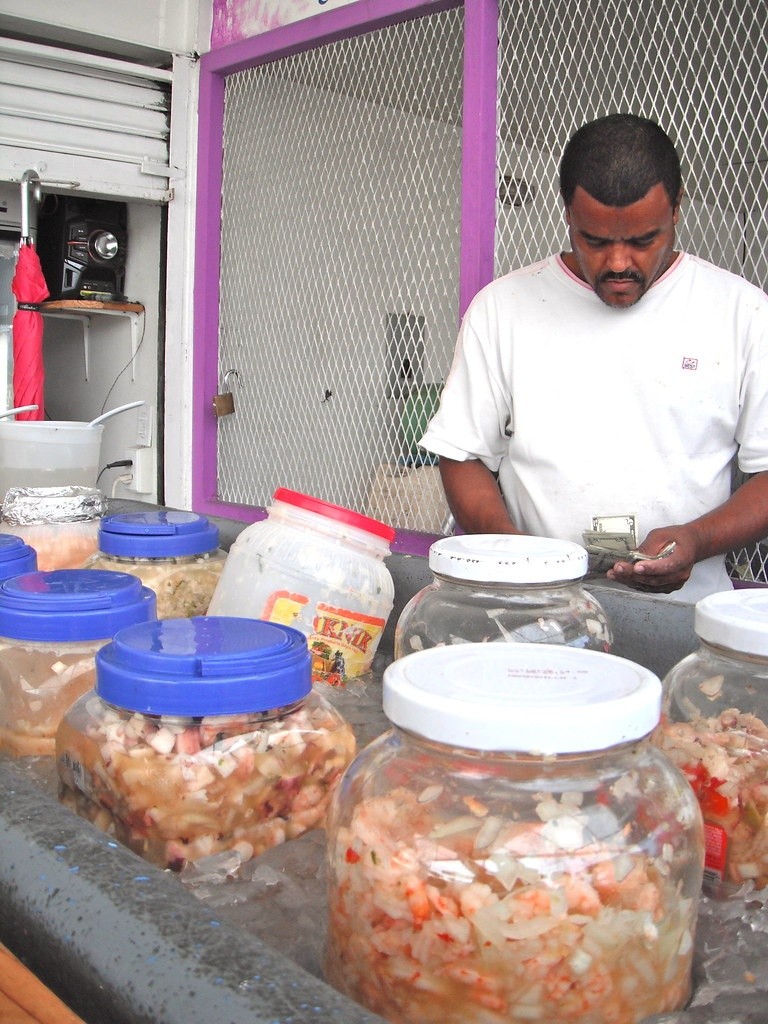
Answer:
[36,192,128,301]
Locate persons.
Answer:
[417,113,768,602]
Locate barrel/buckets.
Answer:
[0,421,104,506]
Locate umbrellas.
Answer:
[11,169,50,420]
